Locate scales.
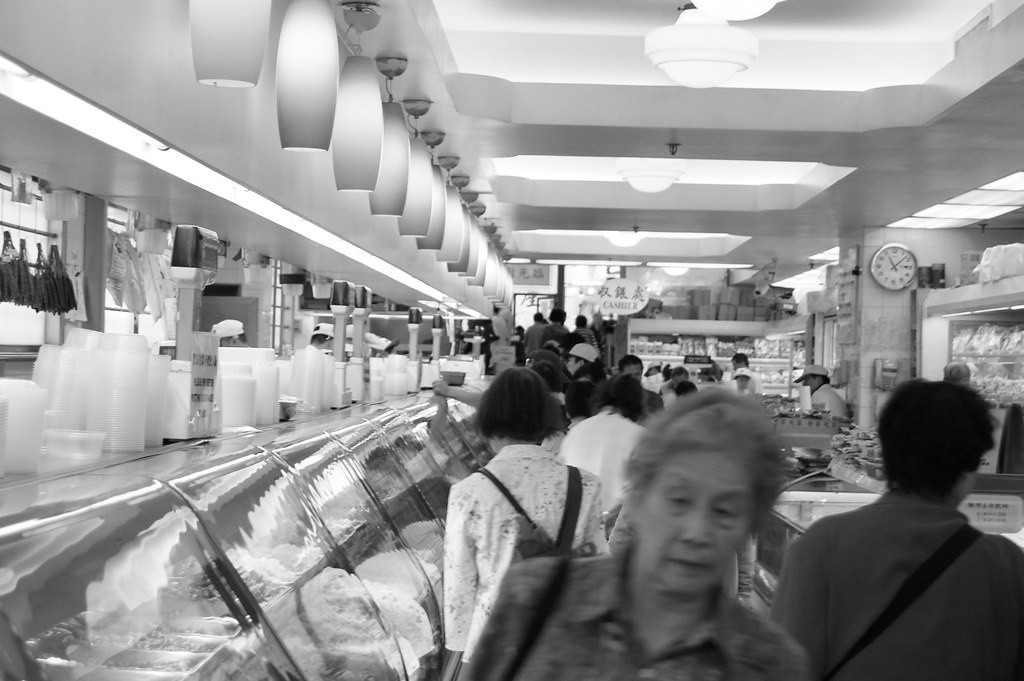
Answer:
[408,307,423,393]
[330,280,357,409]
[346,286,372,403]
[421,315,444,388]
[163,225,224,440]
[439,326,485,379]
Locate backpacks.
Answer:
[473,465,597,561]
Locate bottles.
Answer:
[932,263,945,288]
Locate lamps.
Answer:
[643,0,785,89]
[191,0,516,310]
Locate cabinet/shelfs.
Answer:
[613,317,804,403]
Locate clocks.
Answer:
[870,243,919,290]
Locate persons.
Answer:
[792,365,850,417]
[309,323,400,360]
[441,365,611,681]
[473,304,764,610]
[943,362,971,385]
[212,318,248,348]
[767,379,1024,681]
[457,383,819,681]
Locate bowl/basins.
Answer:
[441,372,465,385]
[216,344,408,427]
[1,328,171,473]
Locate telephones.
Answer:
[874,359,899,391]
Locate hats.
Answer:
[793,364,828,383]
[211,319,245,339]
[560,342,600,362]
[644,361,664,377]
[311,323,334,340]
[732,367,752,379]
